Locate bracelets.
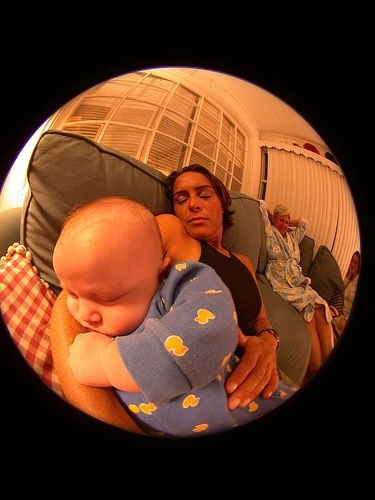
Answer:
[256,328,280,351]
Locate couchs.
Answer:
[0,130,344,385]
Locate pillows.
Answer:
[0,242,66,401]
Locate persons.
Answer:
[332,250,361,341]
[259,199,334,376]
[49,163,281,436]
[52,196,302,438]
